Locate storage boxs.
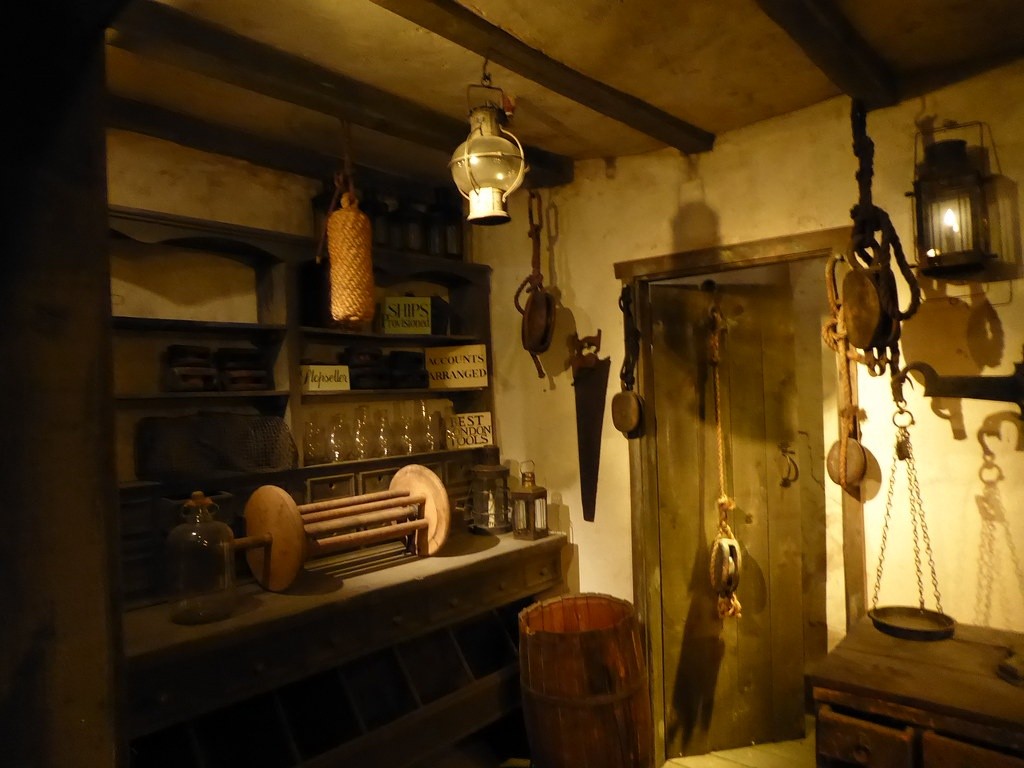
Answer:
[384,295,450,336]
[299,365,350,391]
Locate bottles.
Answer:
[303,398,439,465]
[167,490,235,626]
[470,444,512,536]
[510,459,549,541]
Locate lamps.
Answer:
[510,460,550,541]
[468,443,514,536]
[903,117,998,279]
[448,59,530,227]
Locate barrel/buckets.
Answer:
[517,591,653,768]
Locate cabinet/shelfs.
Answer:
[108,201,568,768]
[802,611,1024,768]
